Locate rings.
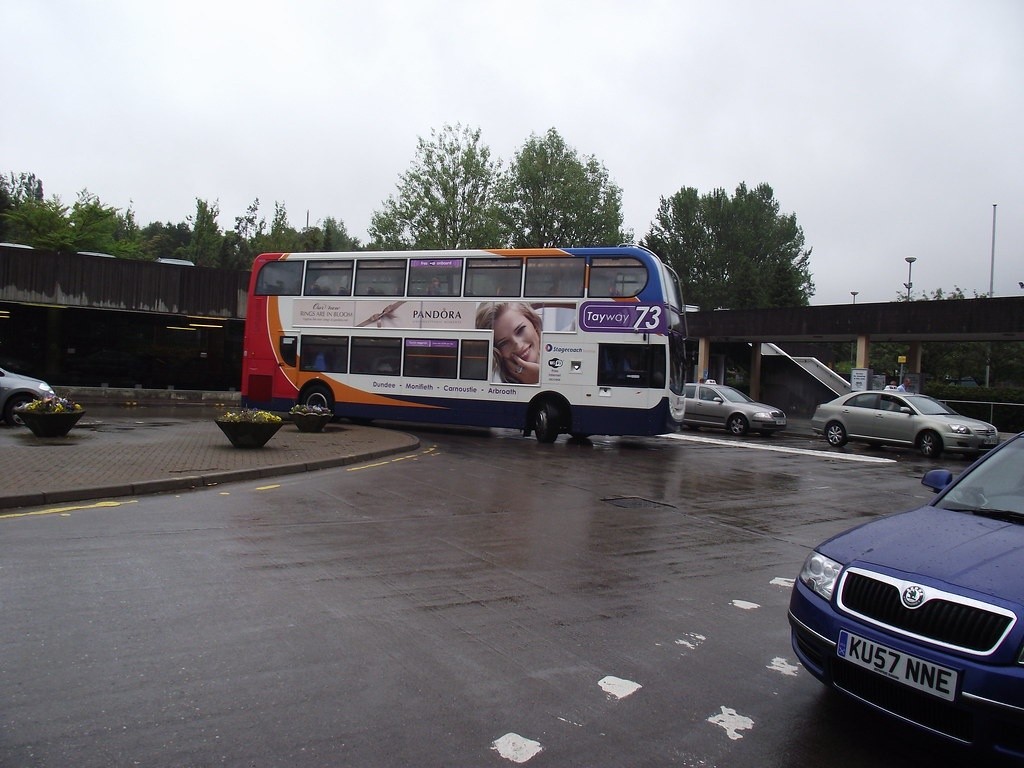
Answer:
[516,366,522,374]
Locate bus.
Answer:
[240,243,698,443]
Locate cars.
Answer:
[787,431,1024,765]
[811,390,999,458]
[0,367,54,427]
[683,383,787,435]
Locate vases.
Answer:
[288,411,334,432]
[13,406,86,438]
[214,418,284,450]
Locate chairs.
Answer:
[267,281,512,297]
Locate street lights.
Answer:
[851,292,859,304]
[904,257,916,301]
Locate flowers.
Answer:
[215,403,282,423]
[291,404,331,415]
[15,395,80,413]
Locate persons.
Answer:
[475,301,542,385]
[885,377,911,410]
[431,276,442,294]
